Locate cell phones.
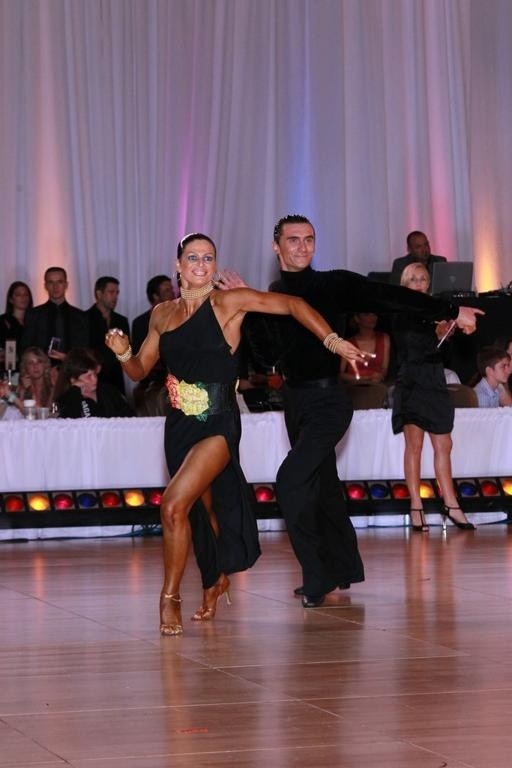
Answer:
[49,337,61,354]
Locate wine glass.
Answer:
[266,363,285,404]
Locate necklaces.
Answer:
[178,280,214,299]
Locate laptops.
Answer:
[431,262,473,295]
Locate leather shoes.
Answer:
[295,582,350,608]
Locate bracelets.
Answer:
[113,344,132,362]
[322,331,344,353]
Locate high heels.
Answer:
[160,595,183,635]
[191,574,231,620]
[410,509,429,532]
[440,504,477,529]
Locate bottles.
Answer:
[23,399,36,419]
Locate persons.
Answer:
[386,262,477,531]
[391,230,448,279]
[213,211,488,608]
[0,265,512,419]
[102,230,379,635]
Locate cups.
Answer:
[36,408,49,419]
